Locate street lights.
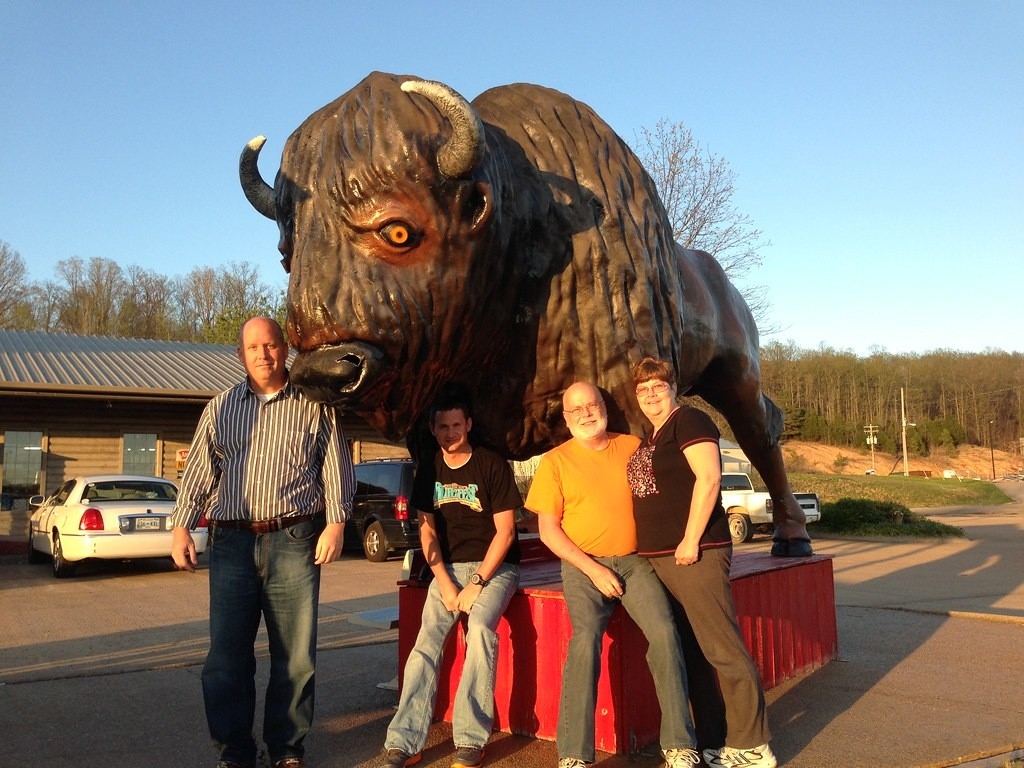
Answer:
[902,422,917,476]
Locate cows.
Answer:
[239,71,813,580]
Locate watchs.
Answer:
[471,574,487,587]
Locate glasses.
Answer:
[565,399,605,416]
[636,382,670,397]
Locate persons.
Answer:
[171,317,357,768]
[382,398,520,767]
[628,358,776,768]
[538,383,696,768]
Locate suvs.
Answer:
[313,456,426,564]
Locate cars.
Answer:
[27,474,210,578]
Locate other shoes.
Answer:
[271,757,305,768]
[216,755,257,768]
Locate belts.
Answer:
[210,516,313,534]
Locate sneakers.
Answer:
[377,746,421,768]
[559,757,586,768]
[451,747,485,768]
[703,743,777,768]
[660,748,700,768]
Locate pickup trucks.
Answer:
[721,471,822,544]
